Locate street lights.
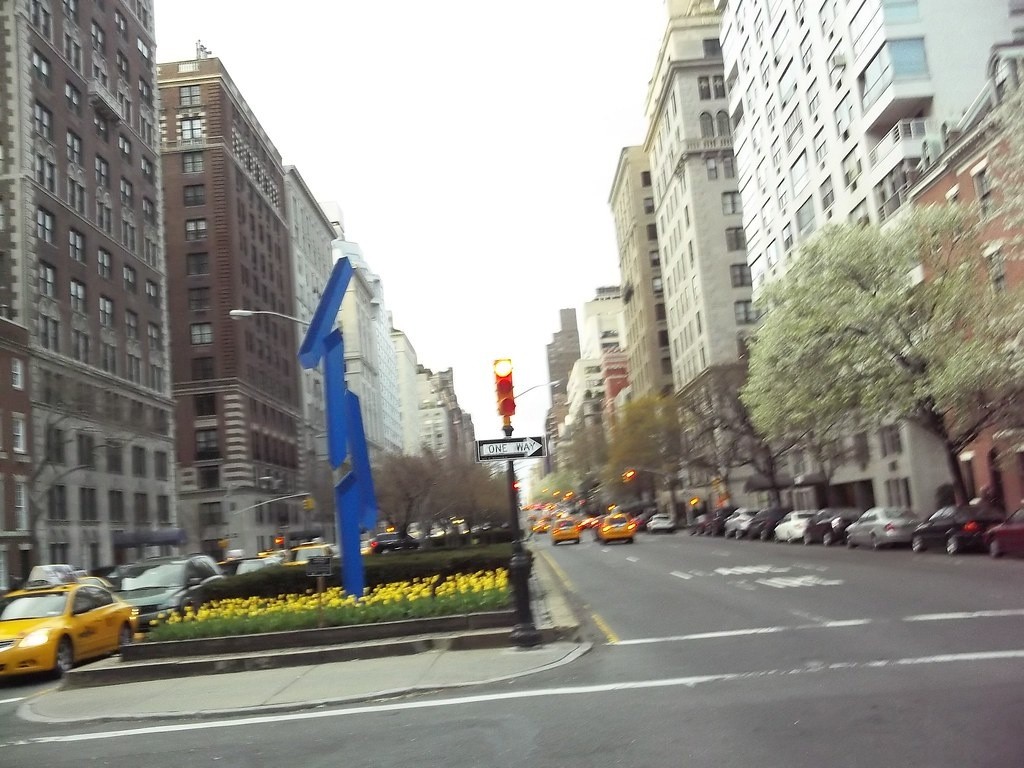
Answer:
[228,307,343,549]
[514,377,562,399]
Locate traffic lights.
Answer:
[275,526,286,547]
[494,358,514,416]
[513,480,522,491]
[626,468,636,481]
[301,498,308,512]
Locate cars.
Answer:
[844,505,923,551]
[369,532,423,555]
[981,504,1024,559]
[0,564,138,676]
[647,514,675,534]
[746,506,794,543]
[214,538,376,579]
[723,507,763,540]
[596,513,636,544]
[803,508,864,547]
[687,506,740,537]
[911,504,1008,556]
[551,517,580,545]
[104,554,228,631]
[518,497,661,534]
[771,510,818,544]
